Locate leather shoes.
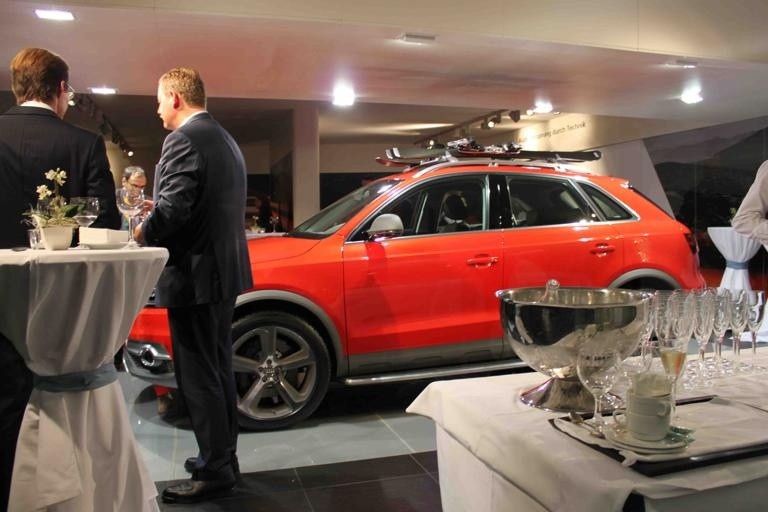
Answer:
[160,457,241,504]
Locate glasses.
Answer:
[66,83,77,100]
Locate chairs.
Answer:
[439,182,584,233]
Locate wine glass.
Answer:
[574,343,624,438]
[69,194,101,251]
[635,285,767,381]
[112,186,147,251]
[495,285,653,415]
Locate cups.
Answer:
[612,388,675,443]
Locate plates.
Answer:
[602,423,691,455]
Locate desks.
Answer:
[425,341,768,512]
[0,243,174,512]
[709,225,761,303]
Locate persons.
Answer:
[116,166,153,230]
[134,67,254,504]
[731,160,768,250]
[0,48,121,248]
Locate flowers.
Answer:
[23,168,87,227]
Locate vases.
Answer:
[40,227,76,251]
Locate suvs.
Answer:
[123,139,707,432]
